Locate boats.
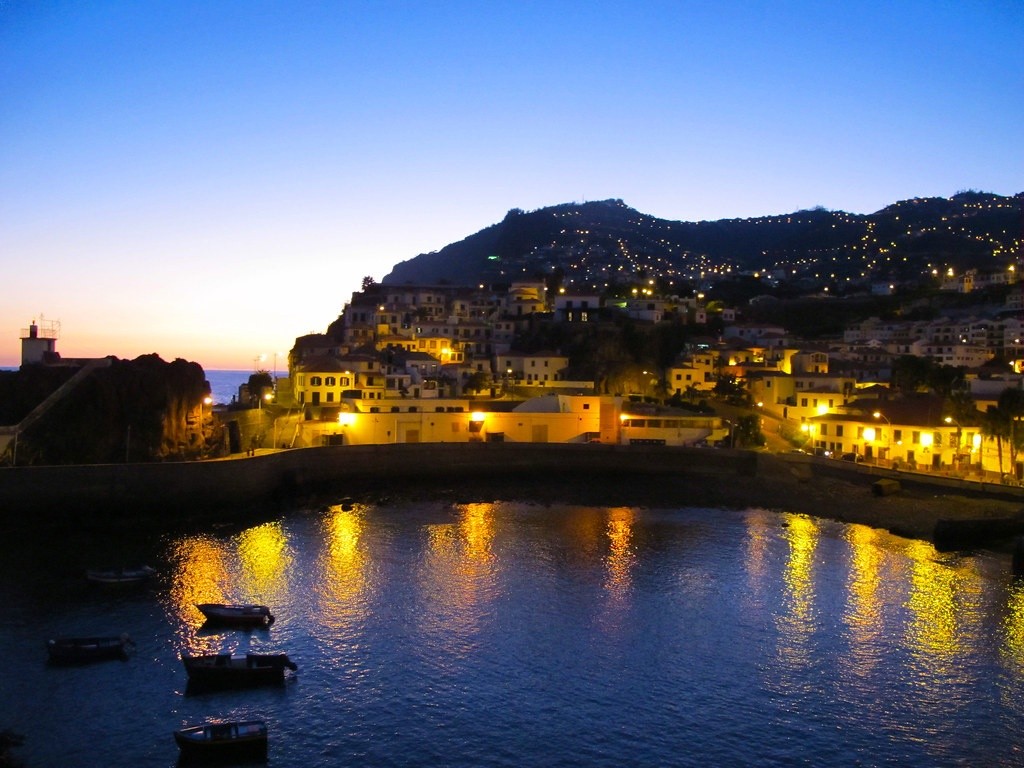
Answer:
[42,633,129,659]
[192,602,275,628]
[172,718,268,753]
[181,653,297,686]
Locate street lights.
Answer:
[872,411,891,466]
[258,394,272,430]
[943,416,966,438]
[199,397,213,432]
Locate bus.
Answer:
[620,415,731,448]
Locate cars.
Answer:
[809,446,834,457]
[840,452,864,464]
